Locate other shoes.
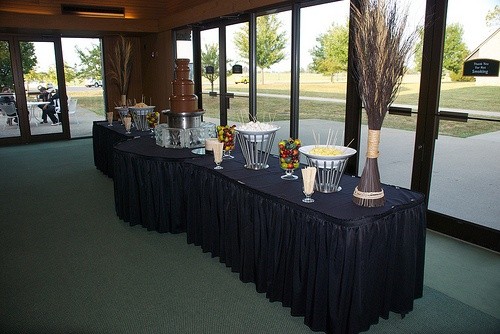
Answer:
[57,122,62,125]
[40,121,48,123]
[51,123,57,126]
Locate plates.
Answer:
[234,125,281,134]
[298,144,357,158]
[128,106,156,109]
[115,107,132,109]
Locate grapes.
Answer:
[291,156,298,162]
[282,146,292,158]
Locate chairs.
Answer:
[52,95,79,126]
[0,103,20,130]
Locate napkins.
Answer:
[124,118,131,128]
[302,166,316,194]
[107,112,113,123]
[213,142,224,162]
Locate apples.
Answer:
[279,138,300,169]
[147,112,159,128]
[217,125,235,150]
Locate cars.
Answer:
[84,77,103,88]
[234,74,249,84]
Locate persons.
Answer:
[37,88,65,125]
[0,86,30,125]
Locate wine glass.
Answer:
[107,112,113,126]
[124,117,132,134]
[301,170,315,203]
[212,142,224,169]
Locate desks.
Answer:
[28,101,51,127]
[93,122,431,334]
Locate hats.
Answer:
[39,87,47,90]
